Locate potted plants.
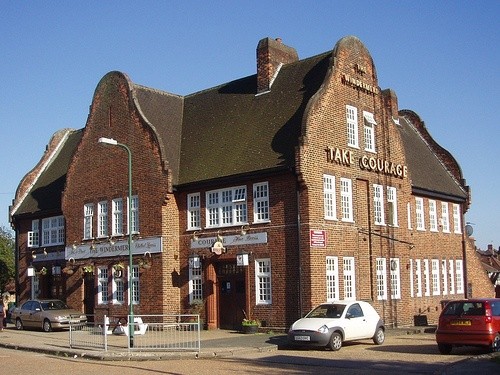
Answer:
[83,265,93,273]
[40,267,49,276]
[190,298,205,314]
[239,319,259,334]
[111,260,127,271]
[136,256,152,270]
[187,318,205,331]
[61,261,74,275]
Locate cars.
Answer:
[435,297,500,353]
[10,299,88,333]
[288,301,386,352]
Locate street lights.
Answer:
[98,137,134,348]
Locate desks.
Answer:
[112,316,127,332]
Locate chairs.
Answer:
[468,307,484,316]
[326,305,337,318]
[350,307,359,315]
[455,305,465,315]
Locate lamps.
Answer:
[71,240,86,250]
[90,236,102,251]
[215,229,224,243]
[31,250,37,259]
[44,247,48,256]
[240,224,249,237]
[128,235,139,245]
[109,234,118,247]
[192,231,199,242]
